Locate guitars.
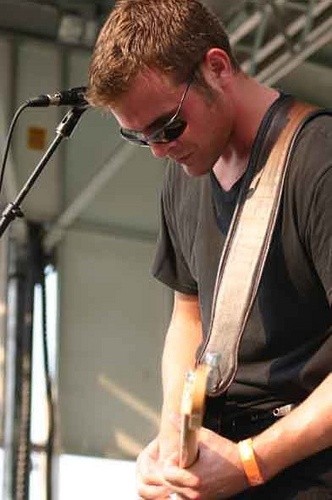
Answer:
[168,364,214,500]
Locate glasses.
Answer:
[120,79,192,148]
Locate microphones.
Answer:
[25,87,91,108]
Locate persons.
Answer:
[85,0,331,500]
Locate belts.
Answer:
[202,409,280,438]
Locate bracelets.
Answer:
[238,437,263,487]
